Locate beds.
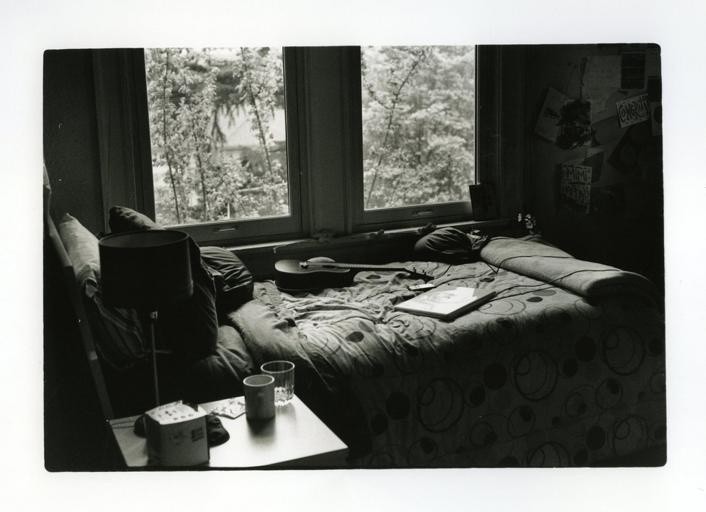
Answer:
[44,212,664,468]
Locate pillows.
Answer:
[55,213,148,356]
[111,206,219,361]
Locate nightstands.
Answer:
[110,388,347,470]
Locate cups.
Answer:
[242,362,295,421]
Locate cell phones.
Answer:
[408,283,436,291]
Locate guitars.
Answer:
[275,257,434,292]
